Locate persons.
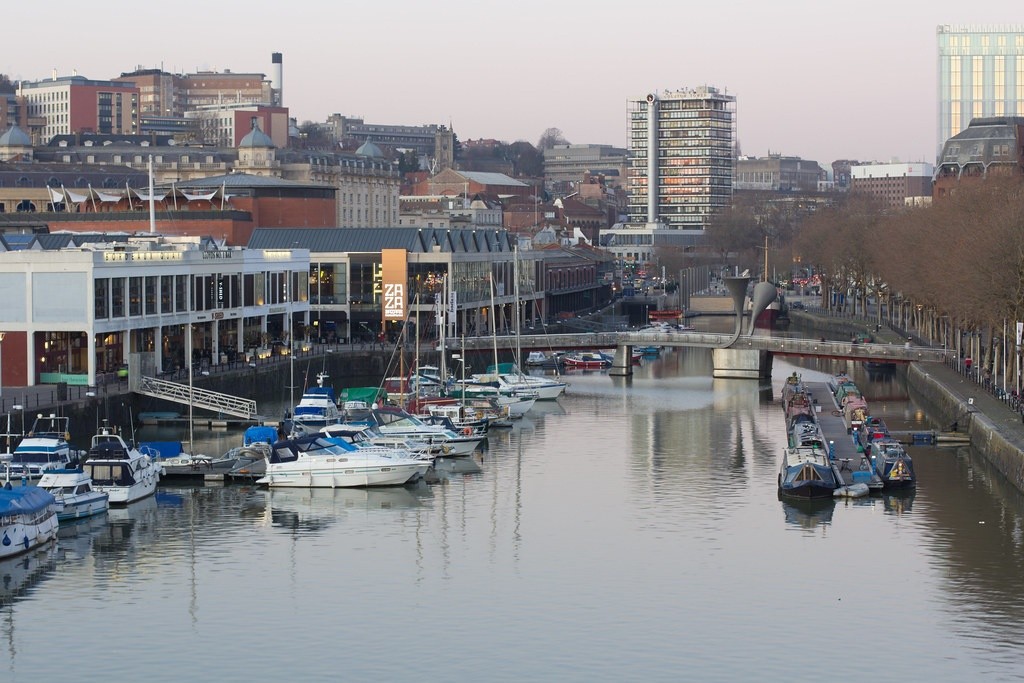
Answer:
[984,369,992,387]
[1010,384,1024,405]
[298,427,306,438]
[964,355,972,372]
[274,429,288,446]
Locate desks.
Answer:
[94,342,290,376]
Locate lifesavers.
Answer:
[464,427,472,436]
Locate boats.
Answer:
[777,373,915,505]
[33,470,108,520]
[0,415,88,489]
[246,319,689,491]
[156,453,238,476]
[78,427,163,502]
[1,485,58,560]
